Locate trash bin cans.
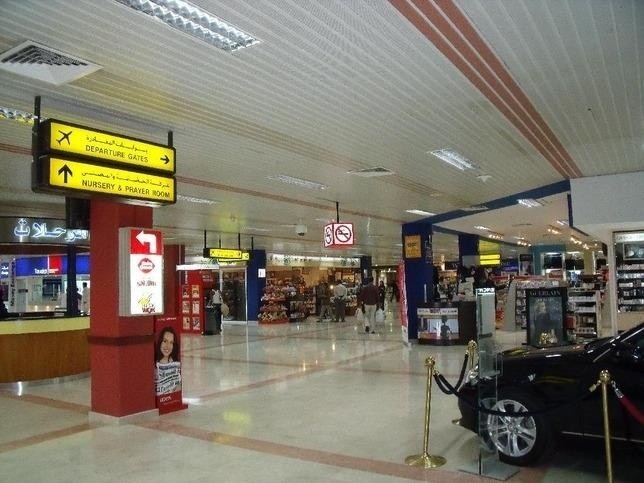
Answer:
[204,305,216,335]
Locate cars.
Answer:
[453,321,643,470]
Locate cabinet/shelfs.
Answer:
[418,228,644,347]
[213,267,362,323]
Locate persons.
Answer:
[157,326,179,363]
[357,276,385,334]
[81,282,90,316]
[333,280,347,322]
[211,287,223,332]
[533,301,559,345]
[486,272,507,308]
[316,283,336,322]
